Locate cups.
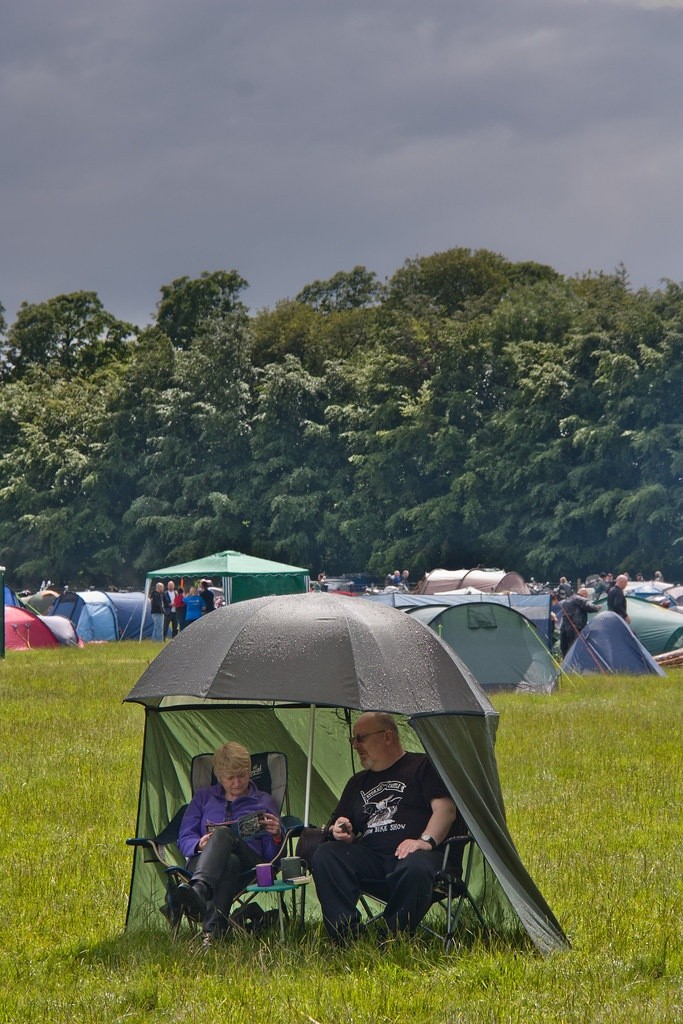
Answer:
[280,856,307,882]
[256,863,273,886]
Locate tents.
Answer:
[558,581,683,676]
[139,550,310,643]
[361,594,560,693]
[411,567,531,595]
[4,583,79,651]
[47,590,173,641]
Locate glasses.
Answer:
[349,730,386,747]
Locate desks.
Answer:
[240,876,309,938]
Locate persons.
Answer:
[150,577,215,641]
[310,574,329,592]
[178,741,287,951]
[534,571,664,658]
[311,712,456,948]
[384,569,408,593]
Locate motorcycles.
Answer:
[528,577,552,595]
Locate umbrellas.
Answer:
[122,587,574,919]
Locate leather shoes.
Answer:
[203,932,213,948]
[176,883,208,922]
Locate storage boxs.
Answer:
[288,875,314,885]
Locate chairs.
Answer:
[324,755,489,953]
[121,749,318,946]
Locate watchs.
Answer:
[417,834,436,850]
